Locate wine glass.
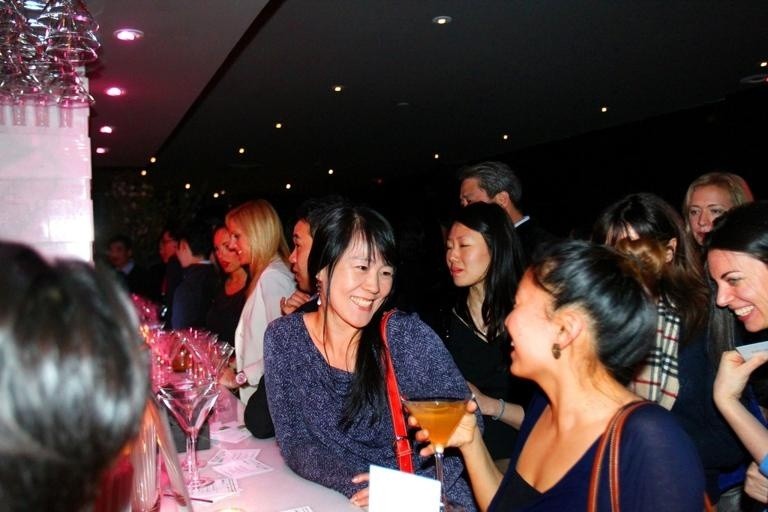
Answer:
[139,324,236,490]
[400,394,476,512]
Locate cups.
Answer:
[0,0,103,110]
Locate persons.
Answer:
[458,159,556,259]
[598,191,768,512]
[104,235,161,301]
[217,200,297,405]
[261,199,488,511]
[0,237,152,512]
[679,170,756,248]
[436,199,537,476]
[708,197,768,512]
[399,237,708,510]
[244,205,341,438]
[204,223,252,354]
[158,223,186,331]
[175,219,221,333]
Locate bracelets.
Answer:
[491,397,505,421]
